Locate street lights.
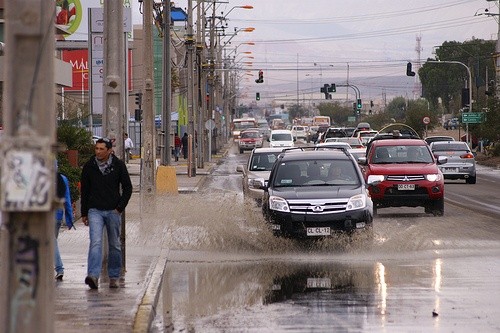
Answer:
[313,62,334,103]
[196,5,256,169]
[305,73,322,118]
[420,97,429,137]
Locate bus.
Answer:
[232,117,256,143]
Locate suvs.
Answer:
[254,145,376,246]
[356,138,447,217]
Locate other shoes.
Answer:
[55,272,63,281]
[109,279,119,288]
[85,276,98,289]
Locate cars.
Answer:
[247,114,378,165]
[443,118,461,130]
[92,135,103,146]
[238,130,263,154]
[430,141,476,184]
[236,147,310,209]
[423,136,455,146]
[313,141,353,178]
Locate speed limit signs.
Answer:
[423,117,431,124]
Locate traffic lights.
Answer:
[256,92,260,100]
[258,70,264,83]
[357,98,362,109]
[135,93,143,105]
[327,83,336,93]
[134,108,143,122]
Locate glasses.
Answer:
[98,138,110,142]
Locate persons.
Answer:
[329,164,346,179]
[54,160,77,280]
[79,138,133,289]
[125,133,135,164]
[174,132,188,162]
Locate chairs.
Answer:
[281,165,301,183]
[259,155,269,169]
[376,147,389,160]
